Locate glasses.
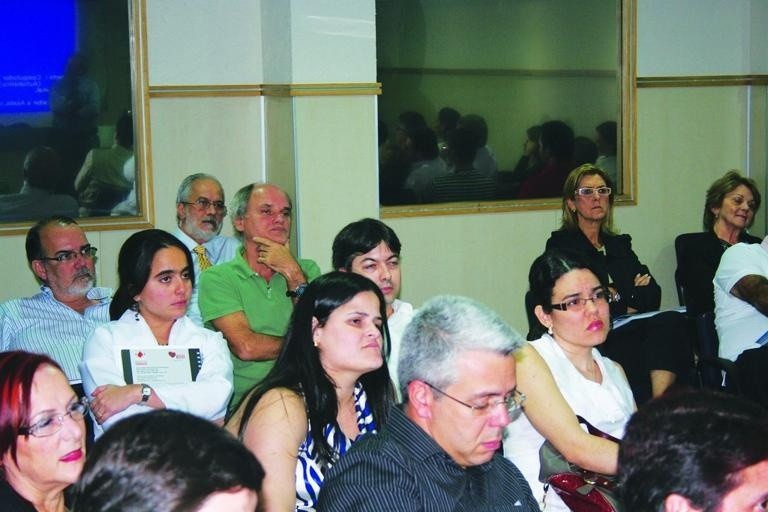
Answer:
[542,291,613,311]
[407,378,526,417]
[573,187,611,197]
[39,247,97,264]
[17,395,92,437]
[180,198,227,217]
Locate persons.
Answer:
[0,110,622,222]
[0,173,417,511]
[317,296,542,512]
[502,163,768,512]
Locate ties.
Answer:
[193,246,213,273]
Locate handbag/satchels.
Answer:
[538,415,623,512]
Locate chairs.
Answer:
[675,231,765,312]
[525,233,632,341]
[694,312,742,399]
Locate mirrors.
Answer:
[0,0,155,237]
[375,0,638,220]
